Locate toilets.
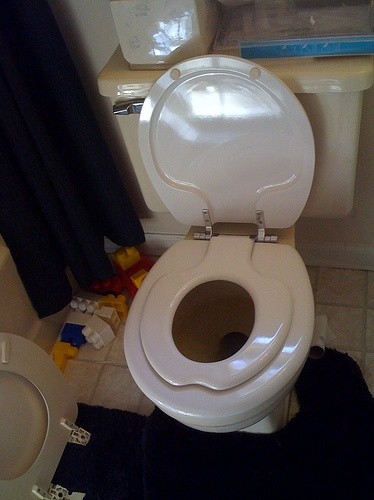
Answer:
[97,44,374,433]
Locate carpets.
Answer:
[42,342,373,500]
